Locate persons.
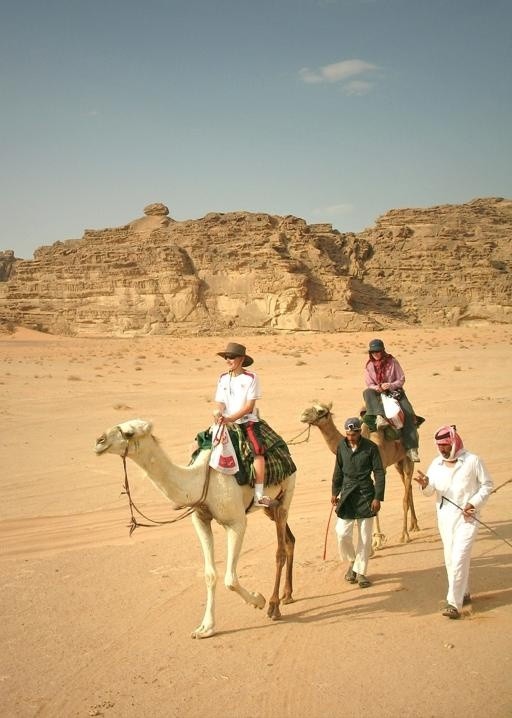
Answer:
[173,343,280,510]
[331,418,385,588]
[413,426,493,619]
[364,340,421,462]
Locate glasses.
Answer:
[222,352,243,361]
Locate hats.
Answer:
[343,416,364,432]
[214,339,256,368]
[367,338,386,353]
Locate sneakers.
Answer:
[406,446,422,463]
[253,494,274,509]
[344,561,357,584]
[373,415,391,432]
[441,604,460,621]
[356,573,371,589]
[463,593,473,606]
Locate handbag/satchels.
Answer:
[208,422,240,476]
